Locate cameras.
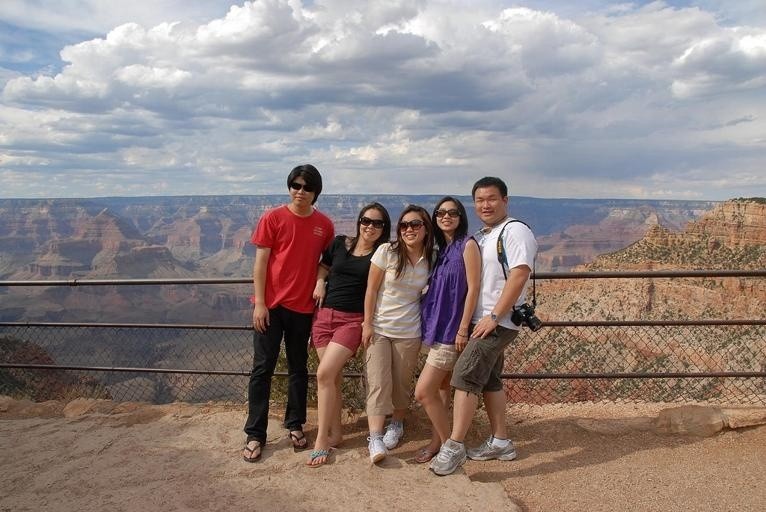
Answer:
[511,302,542,332]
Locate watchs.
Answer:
[490,311,499,323]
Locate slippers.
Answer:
[306,447,334,468]
[288,431,309,451]
[243,442,263,462]
[414,443,439,462]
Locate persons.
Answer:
[413,197,482,464]
[242,164,336,463]
[303,202,390,469]
[428,176,538,477]
[362,204,439,462]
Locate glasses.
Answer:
[359,216,384,229]
[434,208,459,218]
[398,220,425,232]
[290,182,314,192]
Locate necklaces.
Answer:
[357,244,372,256]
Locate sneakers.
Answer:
[429,438,468,476]
[468,433,516,461]
[367,436,386,463]
[383,420,406,449]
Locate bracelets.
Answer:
[457,331,469,338]
